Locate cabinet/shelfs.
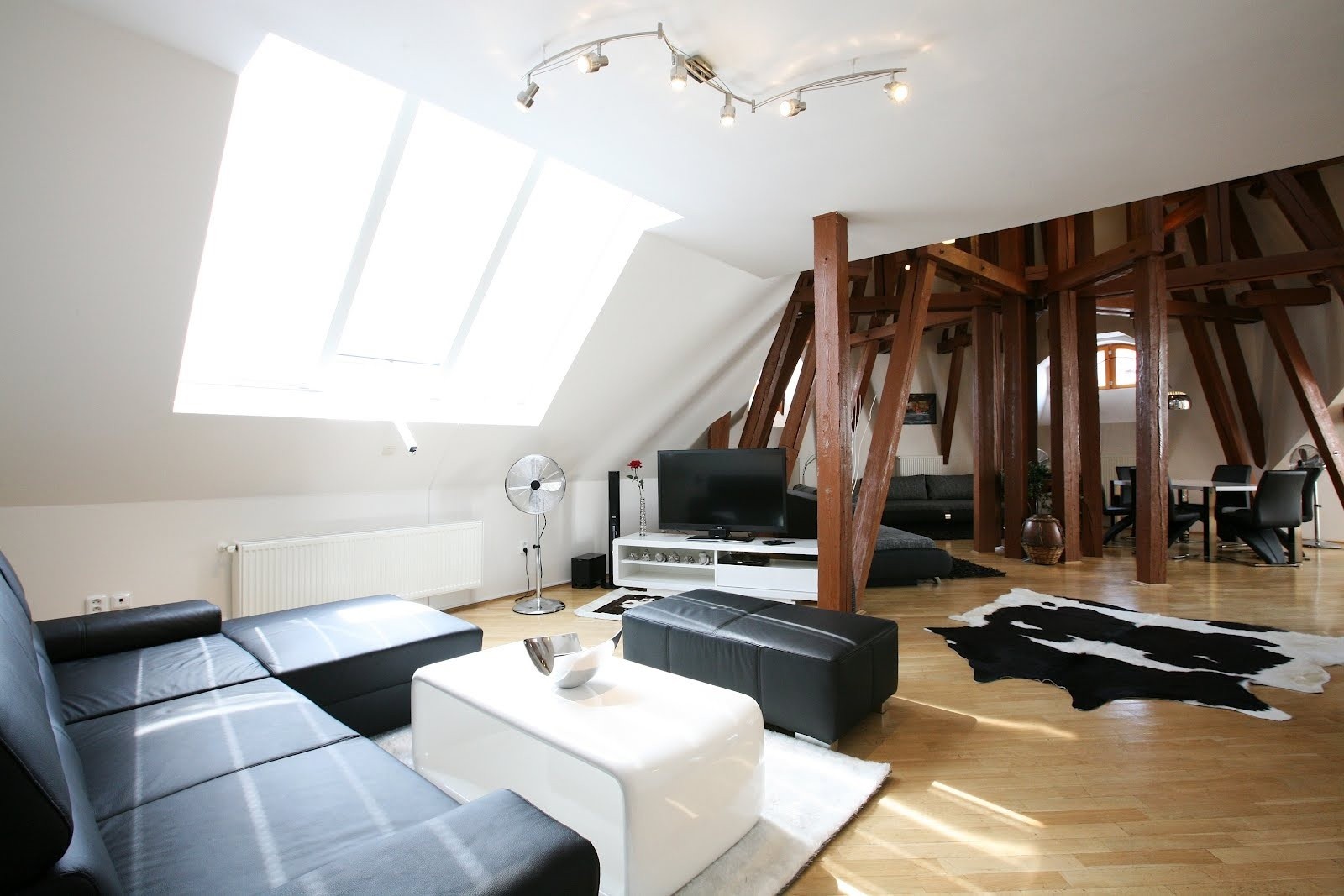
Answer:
[612,532,819,602]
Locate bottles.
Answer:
[1021,514,1063,545]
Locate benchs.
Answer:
[622,588,900,752]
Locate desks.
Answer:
[1110,478,1258,562]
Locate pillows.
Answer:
[925,474,974,499]
[887,474,927,500]
[793,484,817,495]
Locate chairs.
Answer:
[1099,463,1324,568]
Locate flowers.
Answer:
[626,460,644,497]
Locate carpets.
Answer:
[377,554,1344,896]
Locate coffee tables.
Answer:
[410,637,764,896]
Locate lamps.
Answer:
[513,20,914,129]
[1100,357,1192,410]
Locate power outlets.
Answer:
[84,596,109,614]
[518,540,530,555]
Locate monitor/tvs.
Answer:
[658,447,787,545]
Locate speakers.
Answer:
[572,553,607,590]
[601,471,621,589]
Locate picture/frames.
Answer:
[903,393,936,425]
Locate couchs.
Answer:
[788,475,977,585]
[0,550,599,896]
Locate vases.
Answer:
[640,498,647,537]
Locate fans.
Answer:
[1289,444,1343,550]
[504,454,566,614]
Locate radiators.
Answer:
[896,455,944,476]
[222,521,484,619]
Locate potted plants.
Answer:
[1021,459,1064,565]
